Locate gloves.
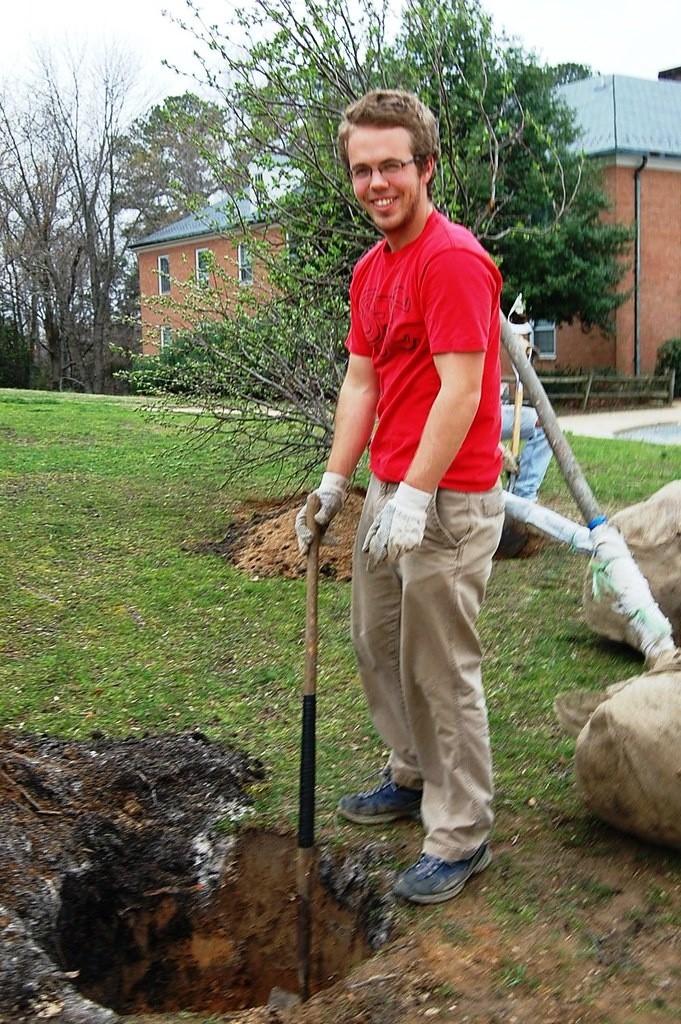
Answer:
[293,471,349,555]
[362,479,434,573]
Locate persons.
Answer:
[294,90,505,905]
[499,333,555,512]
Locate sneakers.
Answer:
[335,778,424,824]
[391,840,492,906]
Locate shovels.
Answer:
[491,376,529,559]
[266,493,322,1011]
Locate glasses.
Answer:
[348,156,425,180]
